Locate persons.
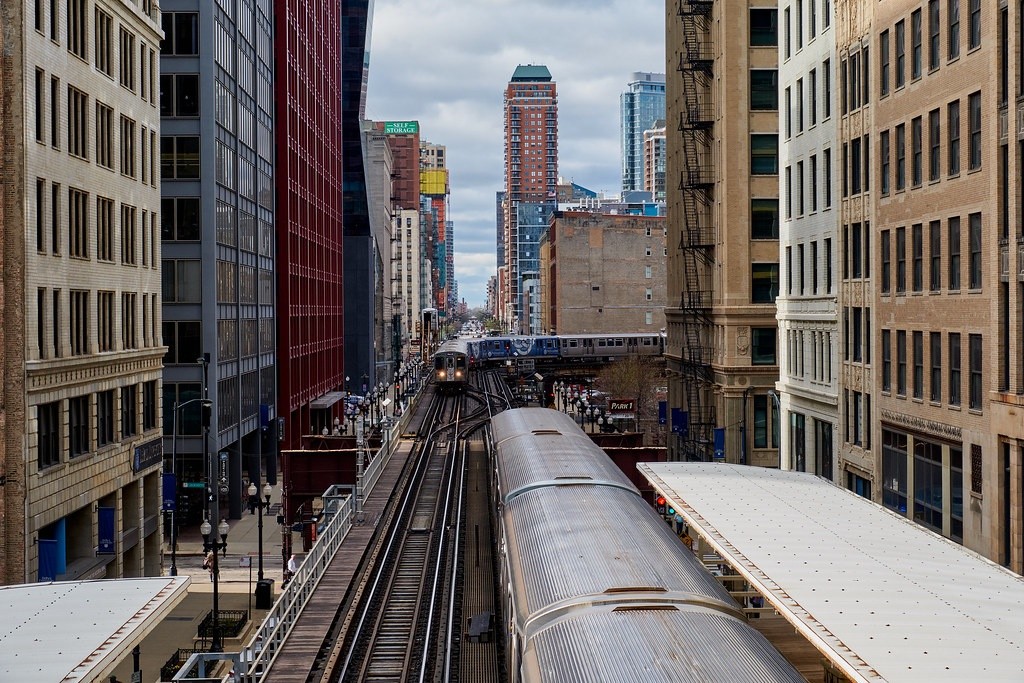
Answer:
[402,401,409,411]
[408,386,412,394]
[673,512,684,535]
[206,550,220,582]
[394,406,402,416]
[749,588,764,619]
[281,554,300,590]
[401,392,405,402]
[108,675,123,683]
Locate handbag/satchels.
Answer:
[203,559,209,570]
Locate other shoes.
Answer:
[281,586,285,590]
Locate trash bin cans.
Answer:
[255,579,275,610]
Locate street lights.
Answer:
[372,386,384,418]
[392,355,425,409]
[365,391,378,428]
[576,398,589,426]
[201,518,230,652]
[378,381,390,416]
[566,391,579,411]
[560,386,571,413]
[345,375,350,409]
[585,405,601,433]
[196,355,212,553]
[597,415,614,434]
[334,415,350,435]
[346,405,361,435]
[169,397,214,575]
[553,380,565,411]
[247,482,272,597]
[357,398,371,435]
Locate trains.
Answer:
[481,406,808,683]
[433,332,668,394]
